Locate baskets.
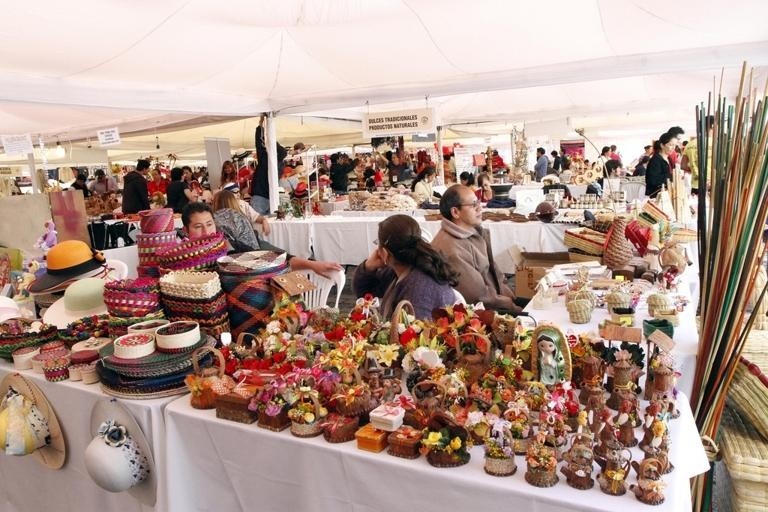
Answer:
[104,231,231,345]
[637,200,669,229]
[0,313,110,360]
[672,227,697,242]
[185,300,533,468]
[597,207,615,222]
[562,226,607,265]
[185,300,492,460]
[723,353,768,438]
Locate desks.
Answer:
[0,186,700,511]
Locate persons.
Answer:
[461,172,495,202]
[534,147,570,182]
[430,184,522,317]
[352,214,459,321]
[122,159,203,214]
[72,174,88,197]
[319,151,415,194]
[482,150,504,173]
[536,331,566,386]
[586,145,621,195]
[632,126,685,198]
[410,154,456,181]
[284,142,306,169]
[210,190,260,250]
[250,112,286,214]
[224,182,271,235]
[181,202,343,280]
[87,169,119,196]
[411,167,440,209]
[221,160,237,185]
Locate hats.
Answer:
[83,398,157,508]
[27,240,128,331]
[223,183,240,192]
[0,373,66,470]
[278,164,309,199]
[0,296,29,324]
[294,143,305,150]
[232,147,252,163]
